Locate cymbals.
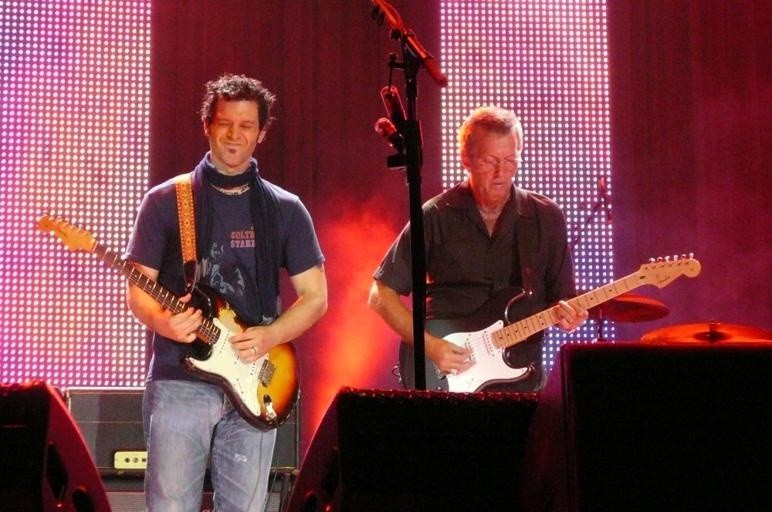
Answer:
[576,290,669,322]
[641,322,772,343]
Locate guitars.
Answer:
[38,215,301,430]
[398,253,702,393]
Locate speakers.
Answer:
[285,388,539,512]
[0,380,112,511]
[541,342,770,510]
[64,387,300,475]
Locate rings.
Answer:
[253,346,260,355]
[451,367,460,374]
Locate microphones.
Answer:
[598,176,613,225]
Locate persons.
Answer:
[124,73,330,512]
[365,106,589,392]
